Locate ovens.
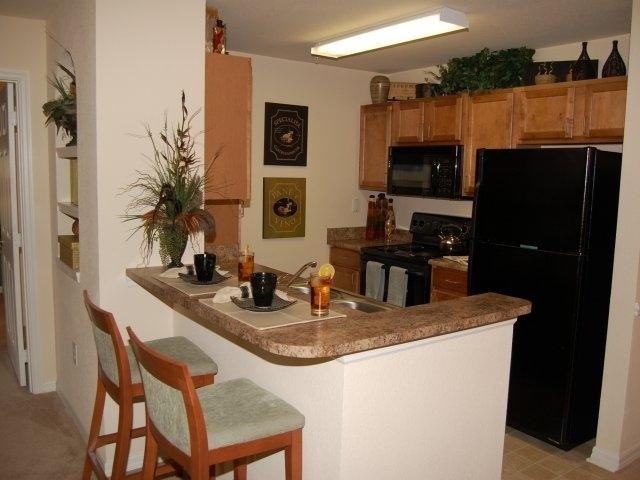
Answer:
[360,251,426,308]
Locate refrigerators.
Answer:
[466,147,622,453]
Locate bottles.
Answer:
[602,39,627,78]
[213,20,227,54]
[572,41,595,81]
[386,198,397,234]
[374,192,389,230]
[366,192,376,230]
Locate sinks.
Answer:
[279,281,348,301]
[331,298,399,315]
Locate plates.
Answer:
[178,268,233,285]
[228,292,297,312]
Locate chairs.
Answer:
[76,283,223,478]
[124,318,309,479]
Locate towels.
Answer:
[212,280,256,303]
[364,260,386,303]
[161,263,200,279]
[387,264,409,309]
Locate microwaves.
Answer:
[387,144,462,199]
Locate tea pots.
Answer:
[433,223,463,245]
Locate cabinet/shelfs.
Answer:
[205,46,255,208]
[359,99,392,196]
[457,88,521,200]
[512,68,629,148]
[389,91,465,150]
[51,141,85,287]
[327,242,361,295]
[428,262,470,306]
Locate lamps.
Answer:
[305,5,475,61]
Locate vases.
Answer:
[60,121,78,148]
[161,225,191,270]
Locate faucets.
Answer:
[285,261,317,287]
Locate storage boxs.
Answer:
[58,232,83,273]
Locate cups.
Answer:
[236,251,255,282]
[250,271,277,308]
[370,75,391,104]
[193,254,217,282]
[308,271,331,317]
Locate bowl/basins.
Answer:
[415,84,442,98]
[535,75,555,85]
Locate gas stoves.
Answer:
[361,242,467,269]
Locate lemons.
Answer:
[318,263,335,282]
